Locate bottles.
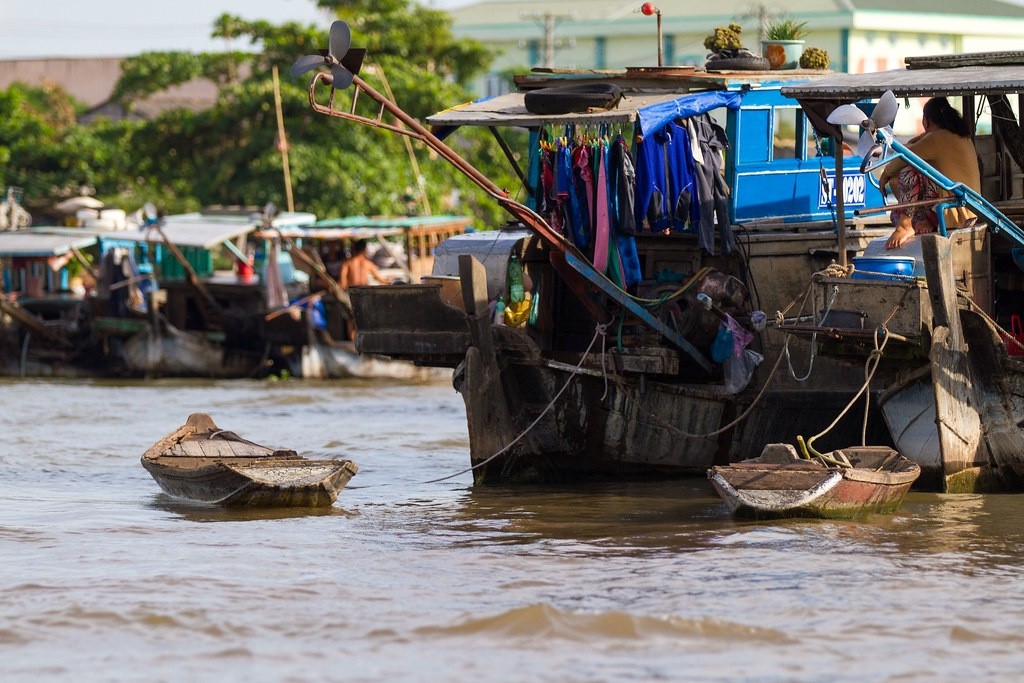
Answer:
[508,255,525,303]
[494,296,506,326]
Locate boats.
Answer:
[141,412,359,507]
[706,447,921,521]
[0,186,477,381]
[289,2,1024,493]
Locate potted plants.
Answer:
[704,23,759,60]
[759,16,811,70]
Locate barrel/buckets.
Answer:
[818,308,868,330]
[849,255,917,283]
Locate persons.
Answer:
[341,239,394,340]
[878,97,982,249]
